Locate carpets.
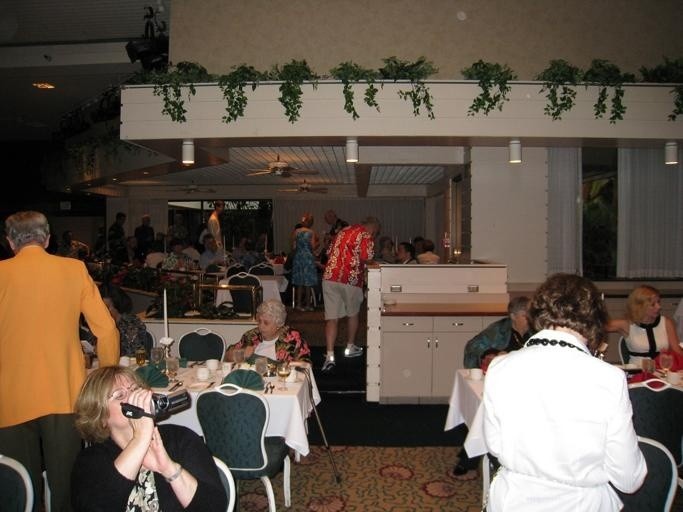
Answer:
[285,308,366,348]
[234,444,497,512]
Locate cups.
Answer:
[118,344,303,393]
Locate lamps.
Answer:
[181,139,195,165]
[508,140,522,163]
[345,138,358,164]
[664,141,679,166]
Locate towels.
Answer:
[627,369,665,389]
[653,349,683,372]
[134,365,169,389]
[219,367,265,392]
[480,357,492,371]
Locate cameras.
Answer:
[149,389,192,425]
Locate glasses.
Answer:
[106,380,140,403]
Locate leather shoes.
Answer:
[452,460,478,475]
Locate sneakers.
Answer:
[344,345,363,358]
[321,354,336,372]
[294,305,315,311]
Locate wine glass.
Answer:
[594,342,682,389]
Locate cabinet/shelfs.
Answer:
[379,316,510,406]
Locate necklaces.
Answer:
[512,334,525,347]
[525,337,586,353]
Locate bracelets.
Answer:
[163,463,185,481]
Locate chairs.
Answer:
[145,247,317,317]
[0,453,34,512]
[144,323,306,512]
[610,377,683,512]
[617,335,630,365]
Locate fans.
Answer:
[272,179,329,194]
[151,180,217,197]
[246,154,320,178]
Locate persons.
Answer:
[0,210,120,511]
[52,200,439,370]
[225,295,312,473]
[71,366,227,512]
[600,284,682,362]
[452,296,537,475]
[485,271,648,512]
